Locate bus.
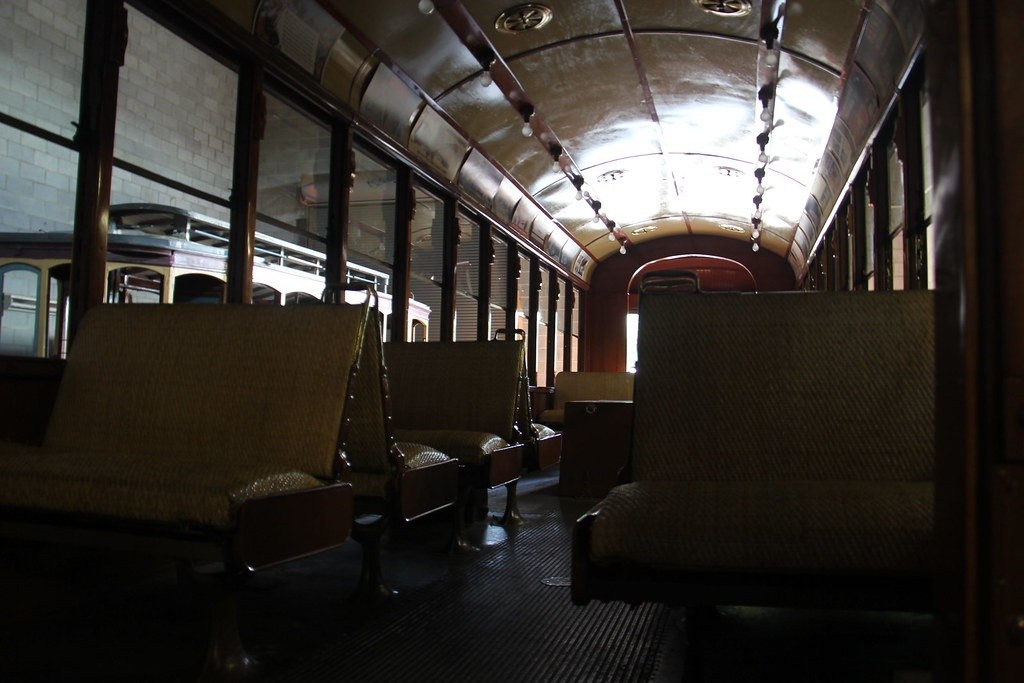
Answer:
[0,202,433,359]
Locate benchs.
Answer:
[568,282,945,656]
[1,297,564,682]
